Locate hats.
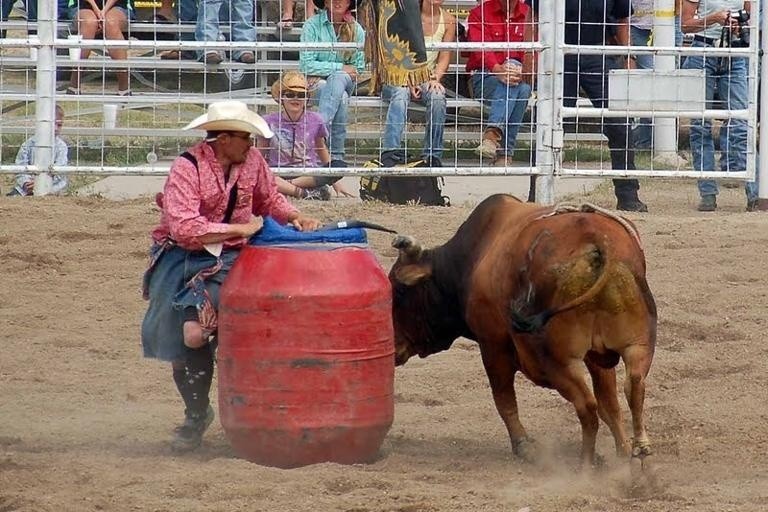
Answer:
[182,101,275,139]
[271,72,318,103]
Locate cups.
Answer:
[103,102,117,130]
[66,33,84,62]
[26,35,39,61]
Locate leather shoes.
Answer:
[161,50,255,64]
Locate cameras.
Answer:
[728,9,750,44]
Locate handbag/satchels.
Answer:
[360,150,451,206]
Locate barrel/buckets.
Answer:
[213,217,395,468]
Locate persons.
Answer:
[140,99,322,452]
[0,0,768,213]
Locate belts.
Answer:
[694,35,743,47]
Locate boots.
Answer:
[474,128,502,159]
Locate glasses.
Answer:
[282,92,305,98]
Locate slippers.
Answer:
[278,19,293,29]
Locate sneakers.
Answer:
[617,197,646,211]
[302,184,332,201]
[175,405,215,450]
[698,195,716,210]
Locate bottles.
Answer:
[145,151,160,164]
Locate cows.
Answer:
[386,192,660,481]
